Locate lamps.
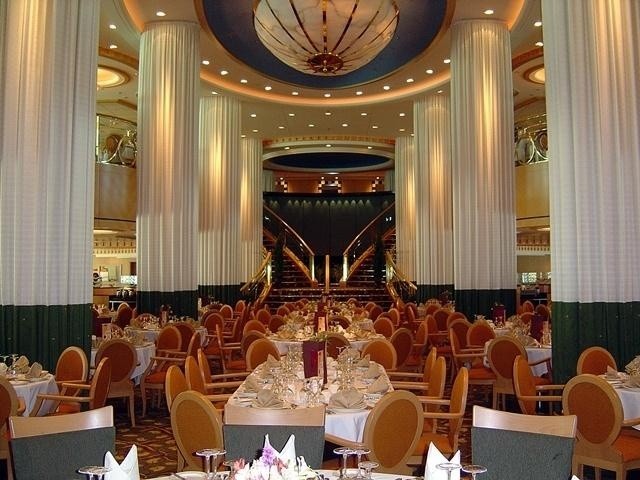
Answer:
[250,0,400,78]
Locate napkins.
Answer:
[16,356,30,372]
[625,374,638,388]
[104,444,141,480]
[606,366,619,379]
[423,442,463,478]
[28,363,42,377]
[263,432,297,468]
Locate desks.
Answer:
[11,373,59,417]
[603,374,639,429]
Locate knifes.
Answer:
[170,472,185,480]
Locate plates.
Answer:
[597,370,640,391]
[170,471,207,480]
[231,359,387,413]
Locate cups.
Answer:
[141,317,160,331]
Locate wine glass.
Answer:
[1,353,20,368]
[77,464,114,480]
[194,446,227,480]
[438,461,487,480]
[333,446,381,480]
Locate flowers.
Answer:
[261,448,290,477]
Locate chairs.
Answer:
[514,353,564,419]
[171,391,226,470]
[576,347,618,376]
[29,358,112,416]
[87,294,554,408]
[54,347,86,413]
[561,374,640,479]
[9,405,114,479]
[223,402,324,469]
[472,404,580,478]
[405,368,469,466]
[1,377,27,428]
[363,390,421,474]
